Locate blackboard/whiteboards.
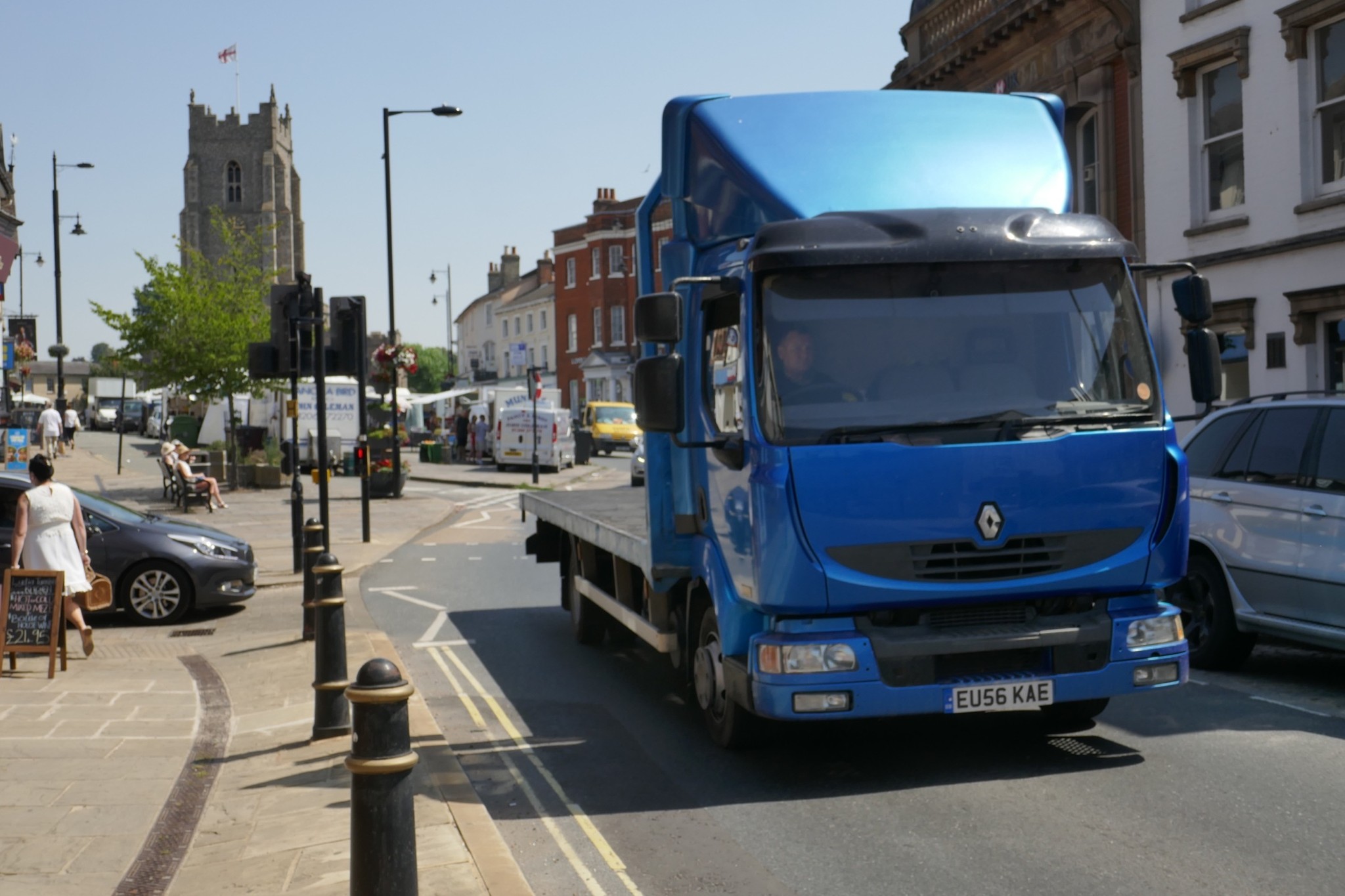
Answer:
[0,568,66,652]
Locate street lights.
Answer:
[48,148,96,451]
[381,105,464,499]
[428,264,455,389]
[14,244,44,320]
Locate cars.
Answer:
[115,399,146,434]
[578,399,644,456]
[1170,386,1345,671]
[626,435,645,487]
[0,470,259,627]
[145,404,162,440]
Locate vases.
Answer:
[361,468,404,498]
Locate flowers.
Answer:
[365,460,411,473]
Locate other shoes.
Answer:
[205,503,217,510]
[53,452,57,458]
[71,444,75,449]
[80,626,94,656]
[217,502,228,509]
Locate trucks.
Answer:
[494,405,576,473]
[489,388,563,463]
[84,376,137,432]
[244,373,362,475]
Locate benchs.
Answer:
[155,458,213,513]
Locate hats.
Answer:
[160,442,176,455]
[176,444,189,455]
[171,438,181,446]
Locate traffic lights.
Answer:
[353,445,371,477]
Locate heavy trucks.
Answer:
[513,86,1222,756]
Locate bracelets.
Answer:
[80,550,89,556]
[11,565,20,570]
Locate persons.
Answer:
[90,406,97,431]
[17,325,30,346]
[37,401,63,460]
[11,454,94,656]
[161,439,229,509]
[456,410,490,464]
[64,403,78,449]
[768,323,861,402]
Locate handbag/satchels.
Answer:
[74,417,81,431]
[57,440,66,455]
[71,564,112,610]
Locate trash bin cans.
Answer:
[427,443,440,462]
[167,416,200,448]
